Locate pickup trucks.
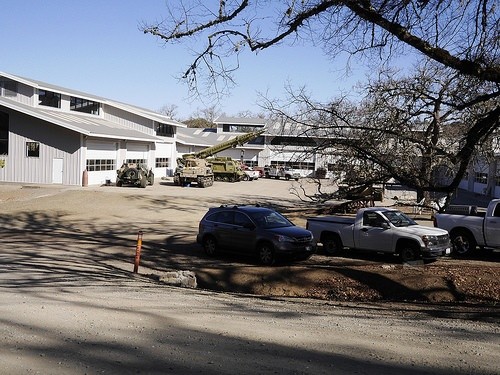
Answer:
[306,206,453,266]
[433,199,500,256]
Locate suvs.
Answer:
[117,163,154,188]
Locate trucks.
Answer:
[262,162,301,182]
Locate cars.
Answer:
[196,205,320,266]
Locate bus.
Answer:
[246,168,261,182]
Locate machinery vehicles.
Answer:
[208,157,247,183]
[174,126,274,188]
[336,176,385,202]
